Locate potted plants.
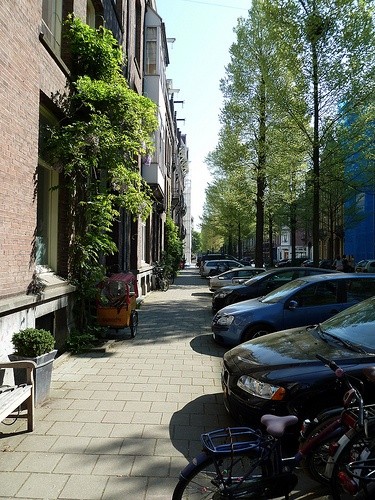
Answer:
[8,327,57,409]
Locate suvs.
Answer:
[275,258,334,269]
[200,261,246,277]
[197,254,243,262]
[355,260,375,273]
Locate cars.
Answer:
[212,270,375,349]
[213,266,336,309]
[221,295,375,423]
[210,267,268,292]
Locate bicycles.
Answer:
[173,352,374,500]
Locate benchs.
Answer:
[0,352,37,432]
